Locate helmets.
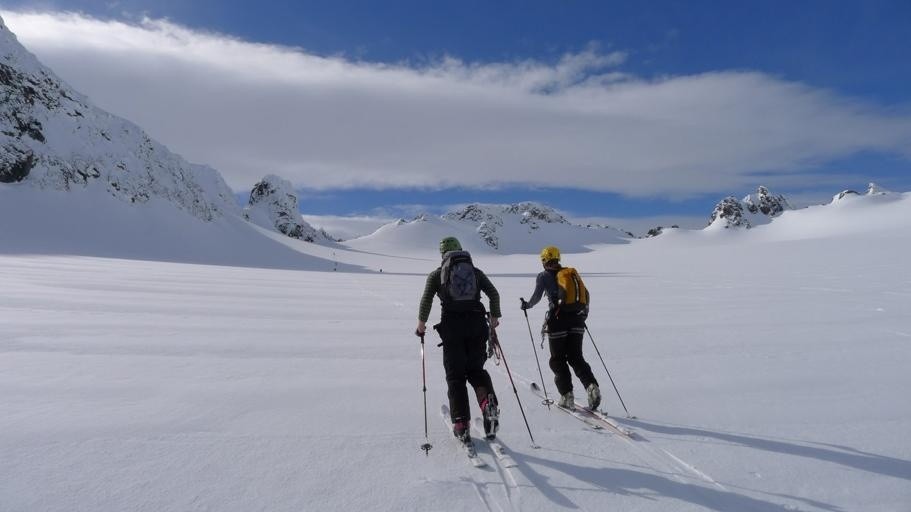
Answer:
[440,237,462,253]
[541,247,560,263]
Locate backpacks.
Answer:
[441,250,481,312]
[557,268,587,312]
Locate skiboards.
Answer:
[531,381,638,438]
[440,402,518,470]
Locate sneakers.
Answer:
[453,421,470,440]
[588,384,601,410]
[559,391,574,407]
[483,404,498,433]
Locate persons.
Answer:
[520,245,602,412]
[415,235,504,443]
